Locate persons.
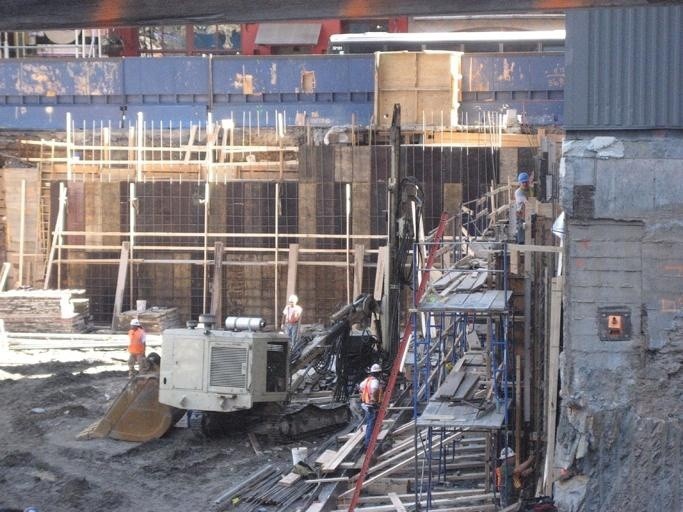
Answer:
[281,294,303,347]
[359,363,383,453]
[495,447,535,506]
[127,318,145,376]
[514,172,532,242]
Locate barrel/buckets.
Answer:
[136,299,147,314]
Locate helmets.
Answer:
[288,294,297,303]
[370,363,382,373]
[498,448,515,459]
[517,172,528,182]
[130,318,139,326]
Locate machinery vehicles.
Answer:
[73,290,373,456]
